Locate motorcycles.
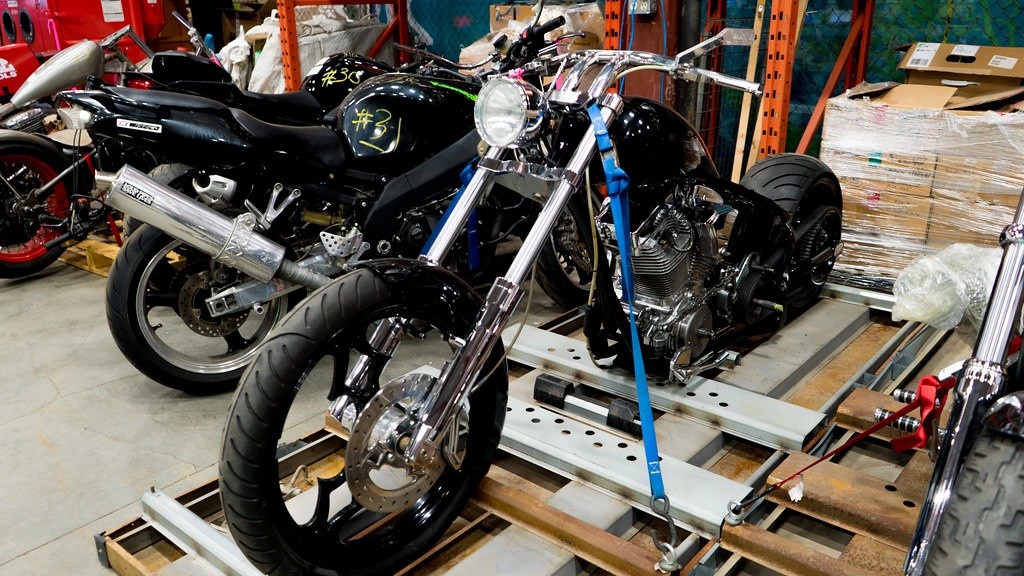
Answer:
[0,0,620,399]
[720,182,1023,576]
[216,27,848,576]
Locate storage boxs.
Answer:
[818,42,1024,281]
[460,4,605,118]
[221,0,277,52]
